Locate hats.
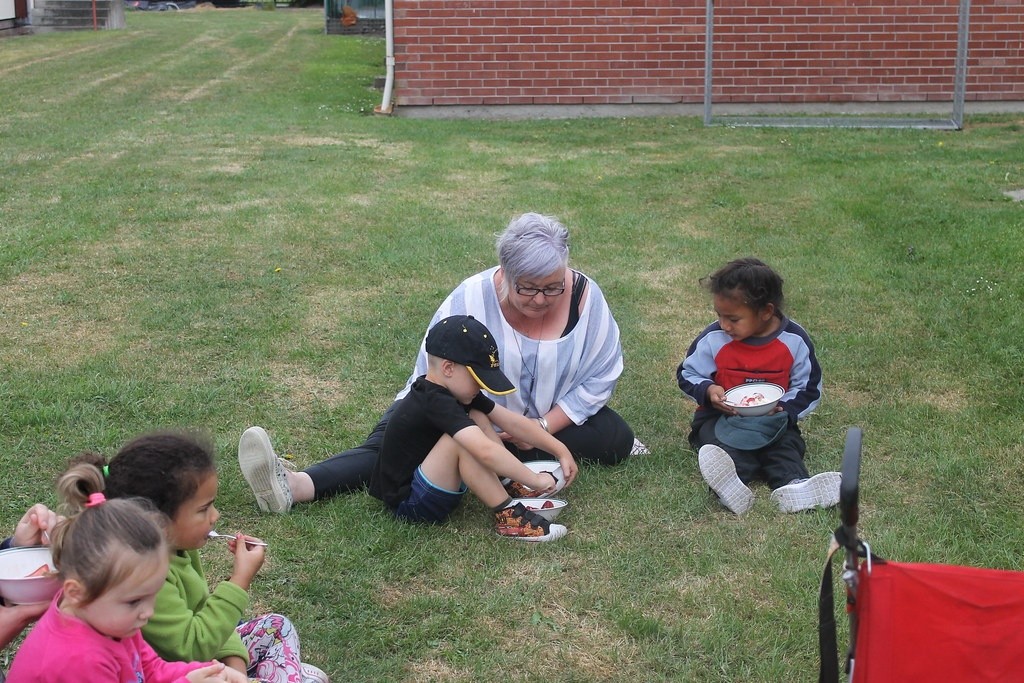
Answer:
[715,411,788,450]
[424,315,516,395]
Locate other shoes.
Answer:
[503,478,557,498]
[776,472,843,514]
[495,503,566,542]
[699,445,753,515]
[301,663,329,683]
[239,426,292,512]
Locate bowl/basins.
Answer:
[513,461,566,497]
[723,383,785,416]
[0,545,63,605]
[511,498,568,522]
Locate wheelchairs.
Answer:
[812,424,1024,683]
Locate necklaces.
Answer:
[506,294,544,417]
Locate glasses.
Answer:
[515,278,565,296]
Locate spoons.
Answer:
[208,530,271,546]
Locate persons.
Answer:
[237,214,634,515]
[87,431,331,683]
[4,465,248,683]
[0,504,69,653]
[676,257,842,517]
[369,315,578,543]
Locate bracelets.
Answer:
[538,416,550,433]
